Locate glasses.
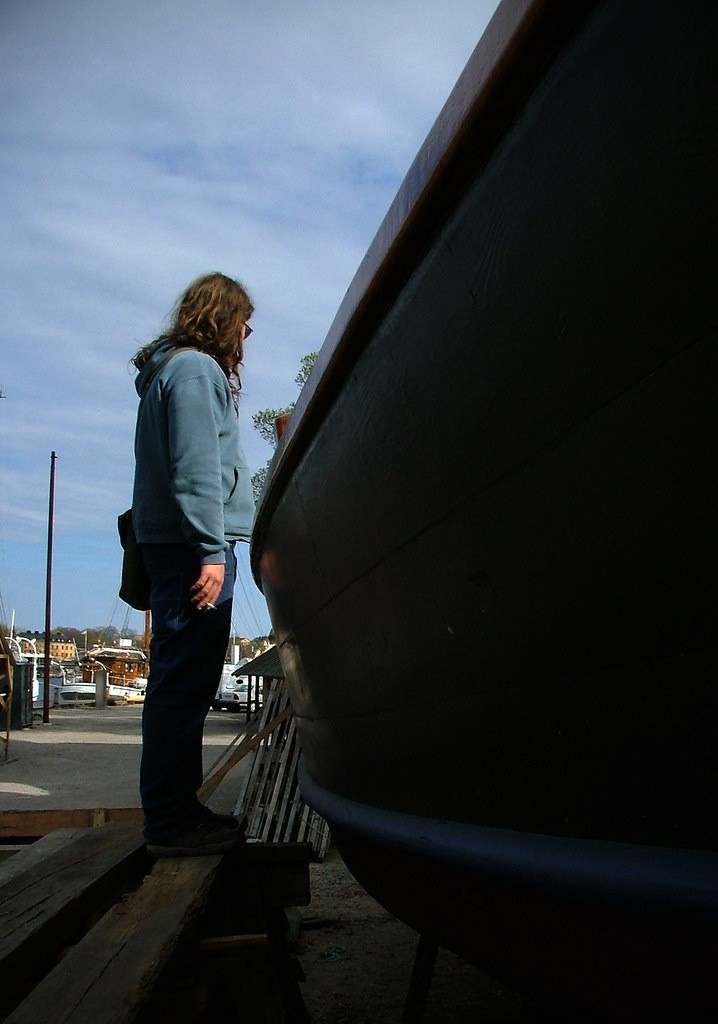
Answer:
[242,321,254,339]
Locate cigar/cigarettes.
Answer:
[207,602,217,609]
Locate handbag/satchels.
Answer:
[118,509,152,610]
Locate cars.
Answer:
[221,684,264,714]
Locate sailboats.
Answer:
[54,597,153,706]
[212,539,273,708]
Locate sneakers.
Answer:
[145,807,247,857]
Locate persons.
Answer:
[116,270,258,858]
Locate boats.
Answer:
[249,0,718,1024]
[0,592,61,702]
